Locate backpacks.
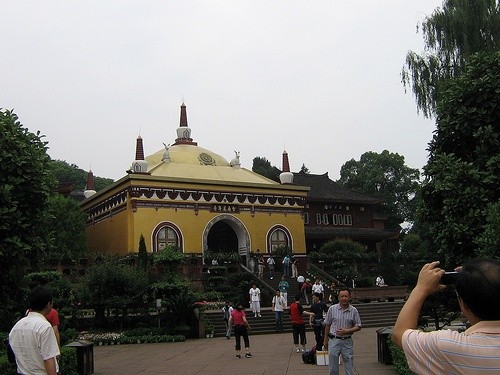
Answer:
[306,282,311,295]
[223,307,230,326]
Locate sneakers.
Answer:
[245,353,253,358]
[296,349,300,352]
[236,354,241,359]
[302,348,310,353]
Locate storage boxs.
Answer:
[316,351,340,366]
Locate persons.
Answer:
[376,275,385,287]
[255,248,324,306]
[222,301,233,339]
[272,289,287,333]
[249,282,262,317]
[288,296,316,352]
[324,289,363,375]
[228,301,253,359]
[8,285,62,375]
[391,257,500,375]
[309,292,329,343]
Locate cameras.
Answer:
[438,271,462,285]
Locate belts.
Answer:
[333,336,351,339]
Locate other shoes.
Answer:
[226,335,231,339]
[254,314,261,317]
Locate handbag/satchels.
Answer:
[7,344,15,363]
[314,317,324,326]
[302,351,315,364]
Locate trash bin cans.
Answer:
[65,339,95,375]
[376,326,393,364]
